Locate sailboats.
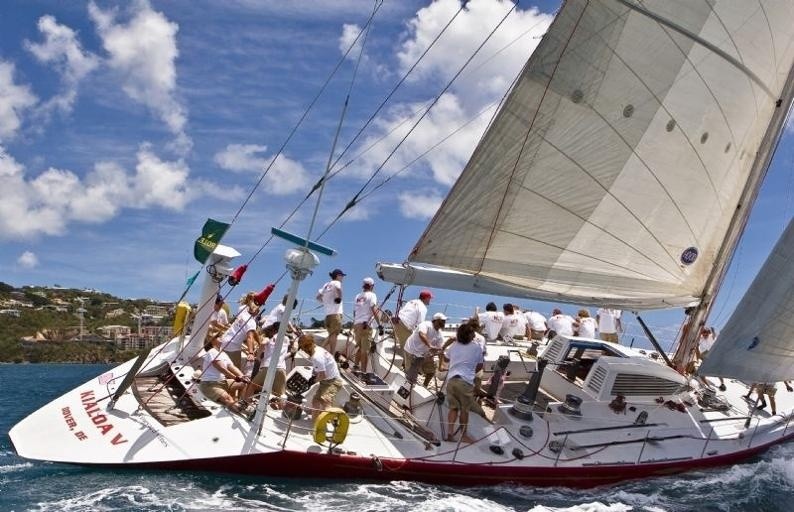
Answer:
[9,1,794,492]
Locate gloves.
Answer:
[309,378,315,385]
[335,298,341,303]
[379,326,384,336]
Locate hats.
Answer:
[329,269,347,278]
[432,313,448,321]
[363,278,374,288]
[420,290,434,300]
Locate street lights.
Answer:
[75,307,87,341]
